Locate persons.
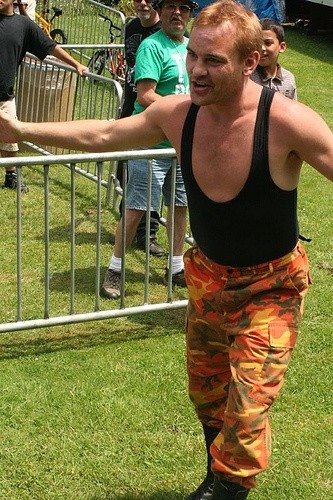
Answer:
[0,0,333,500]
[0,0,89,193]
[249,18,298,103]
[101,0,199,300]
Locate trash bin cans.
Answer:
[14,52,77,153]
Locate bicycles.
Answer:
[86,14,127,86]
[35,6,67,51]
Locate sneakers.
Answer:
[102,265,125,299]
[4,173,29,193]
[164,267,186,287]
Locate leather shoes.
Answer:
[137,234,164,256]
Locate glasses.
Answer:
[164,3,192,15]
[134,0,153,4]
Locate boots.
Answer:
[186,426,219,500]
[200,476,249,500]
